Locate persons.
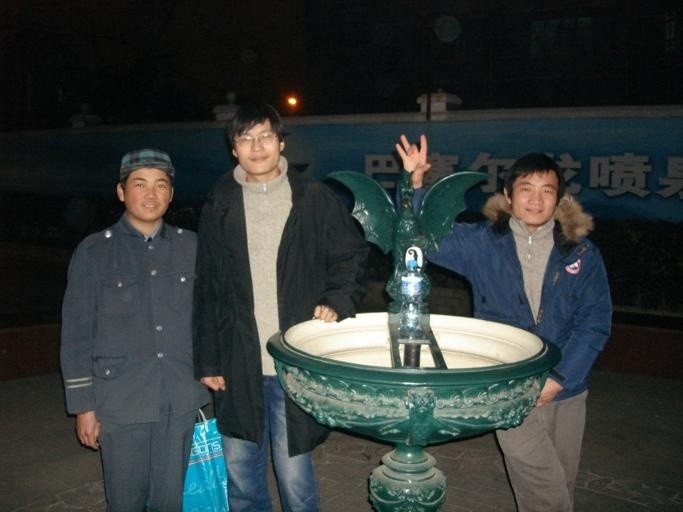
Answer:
[190,101,372,512]
[396,133,613,512]
[60,149,212,511]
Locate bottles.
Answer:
[399,259,425,339]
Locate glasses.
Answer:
[239,132,276,146]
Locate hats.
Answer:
[119,148,176,182]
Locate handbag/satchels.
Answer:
[182,408,230,512]
